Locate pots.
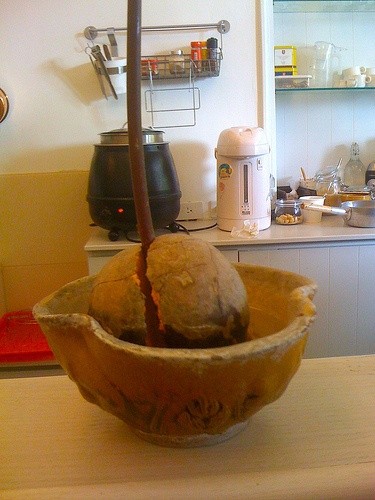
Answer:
[300,200,375,228]
[99,121,163,144]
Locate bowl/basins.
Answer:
[338,191,370,202]
[32,260,320,451]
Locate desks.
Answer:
[0,354,375,500]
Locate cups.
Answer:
[337,65,375,87]
[95,56,127,95]
[300,195,326,223]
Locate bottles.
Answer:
[365,160,375,200]
[313,166,341,216]
[297,178,316,196]
[168,49,185,74]
[344,143,366,187]
[190,37,218,73]
[274,197,304,225]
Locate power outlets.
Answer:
[178,201,202,220]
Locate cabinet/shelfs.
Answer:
[89,239,375,358]
[273,0,375,93]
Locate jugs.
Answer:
[310,40,342,88]
[217,125,272,233]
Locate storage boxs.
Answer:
[273,45,298,76]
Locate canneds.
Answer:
[188,41,208,74]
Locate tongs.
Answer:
[92,45,119,100]
